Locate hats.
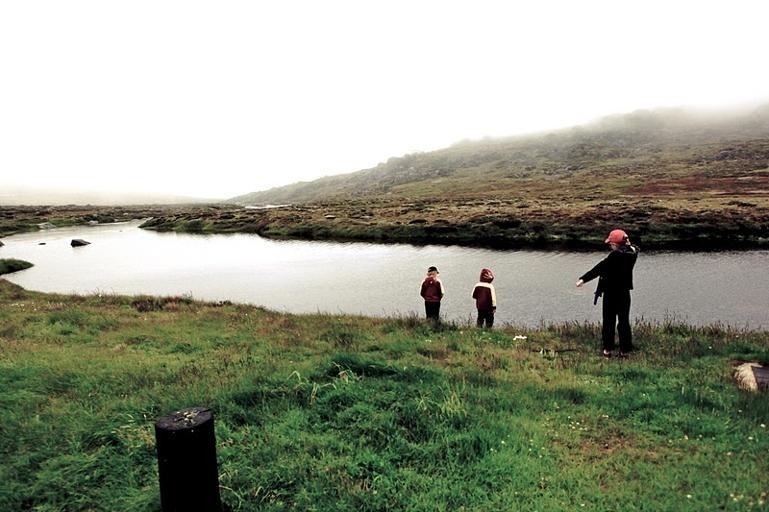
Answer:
[428,267,439,273]
[604,229,629,244]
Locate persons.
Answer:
[575,229,640,357]
[421,266,445,322]
[471,268,496,332]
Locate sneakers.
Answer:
[604,344,640,359]
